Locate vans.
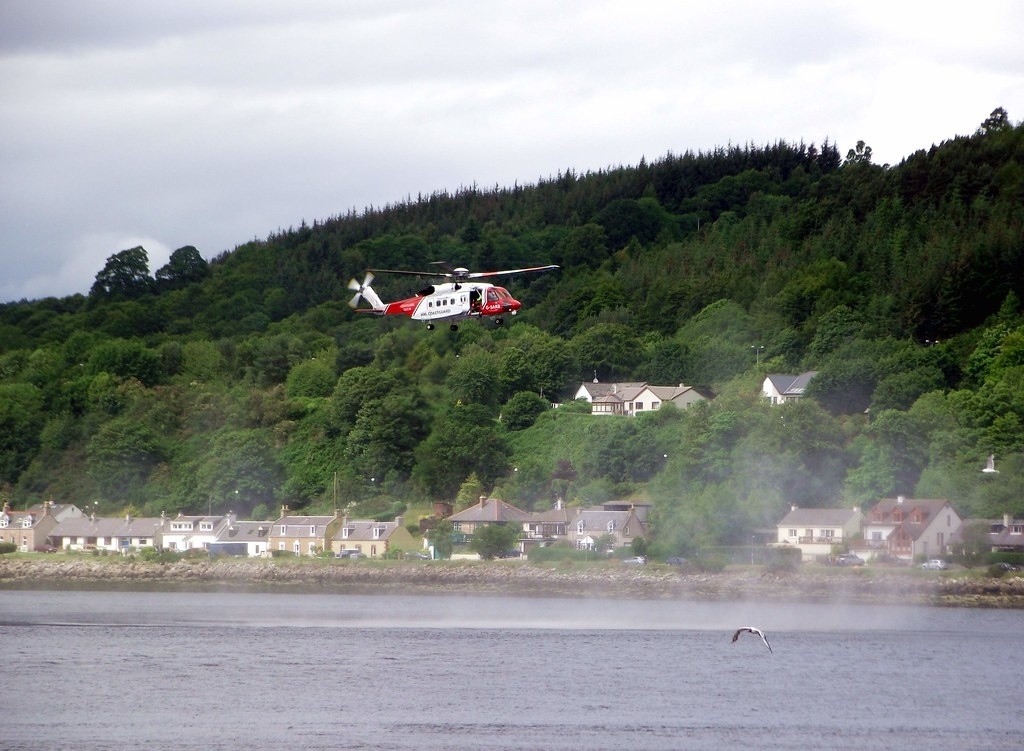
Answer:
[340,549,360,559]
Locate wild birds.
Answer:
[730,627,773,654]
[981,453,1000,473]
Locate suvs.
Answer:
[921,559,947,570]
[624,556,647,564]
[405,552,428,560]
[835,554,864,566]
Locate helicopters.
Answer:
[347,261,561,331]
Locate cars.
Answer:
[995,563,1018,571]
[497,549,522,558]
[666,557,686,565]
[33,544,57,553]
